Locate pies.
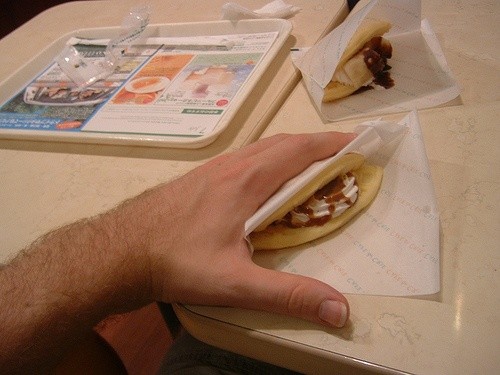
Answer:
[248,151,383,251]
[323,18,392,102]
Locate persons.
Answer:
[0,127,369,375]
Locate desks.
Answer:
[171,0,498,374]
[1,0,348,261]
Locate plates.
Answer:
[126,75,169,94]
